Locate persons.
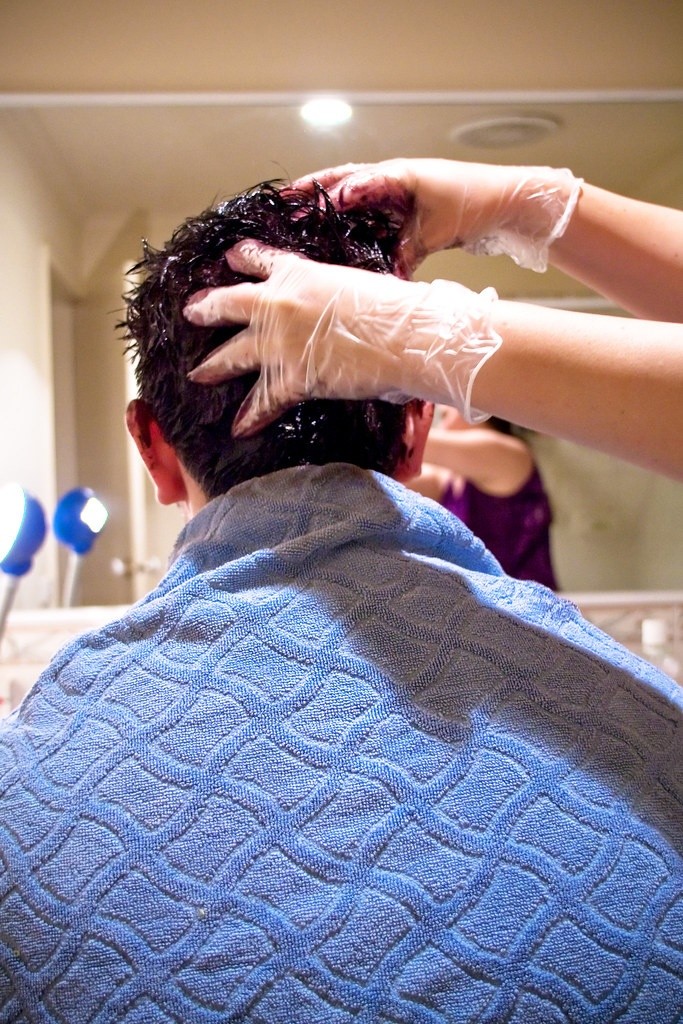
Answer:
[183,157,683,480]
[407,399,559,594]
[0,176,683,1024]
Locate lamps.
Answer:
[0,490,46,644]
[52,485,108,607]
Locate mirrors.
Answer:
[0,90,683,627]
